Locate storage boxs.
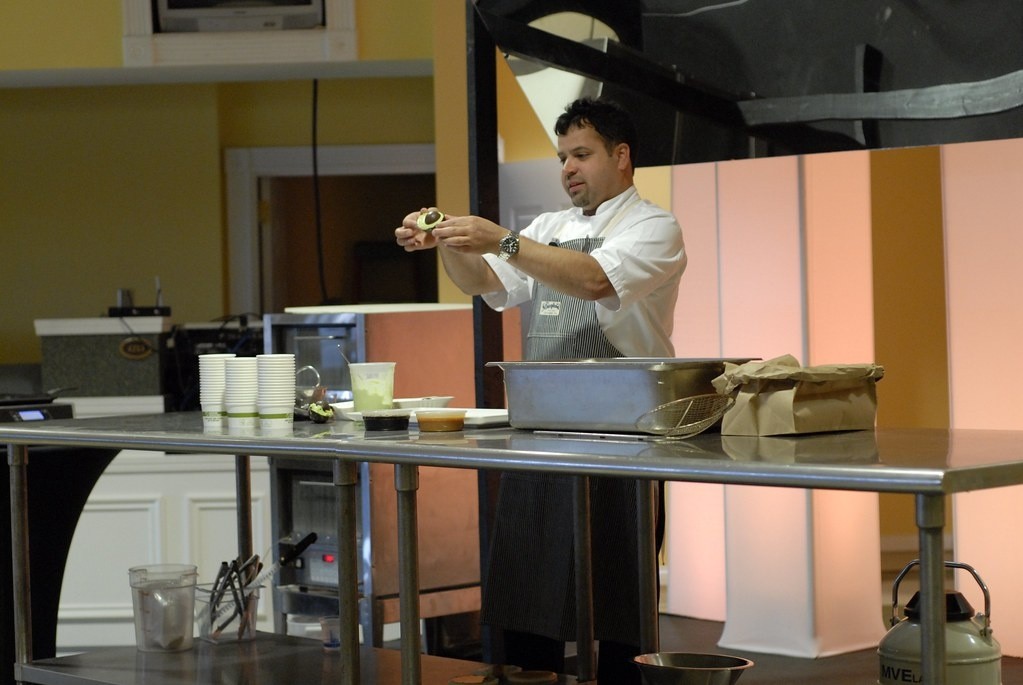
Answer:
[484,353,760,438]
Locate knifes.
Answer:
[213,531,319,622]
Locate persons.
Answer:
[393,95,692,685]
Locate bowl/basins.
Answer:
[632,653,755,684]
[198,352,296,431]
[414,408,468,431]
[360,409,411,432]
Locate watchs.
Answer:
[496,227,522,263]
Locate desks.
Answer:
[0,404,1023,685]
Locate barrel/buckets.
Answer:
[876,557,1003,683]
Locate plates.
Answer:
[392,396,455,411]
[329,401,355,420]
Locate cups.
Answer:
[319,613,340,654]
[194,578,267,645]
[126,563,198,654]
[346,362,396,412]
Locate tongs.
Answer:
[196,554,265,642]
